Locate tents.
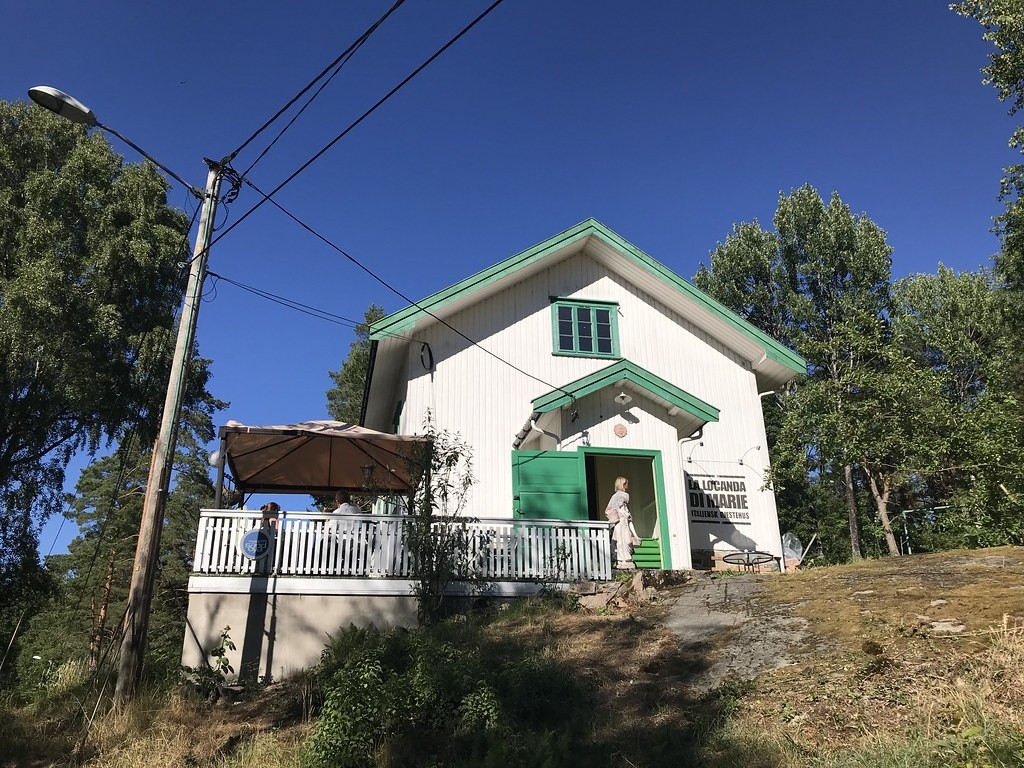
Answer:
[211,415,438,519]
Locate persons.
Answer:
[604,476,642,567]
[258,501,282,528]
[324,488,363,532]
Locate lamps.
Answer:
[686,440,705,462]
[614,392,632,405]
[738,445,760,466]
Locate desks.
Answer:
[724,552,774,575]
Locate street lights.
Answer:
[26,81,219,703]
